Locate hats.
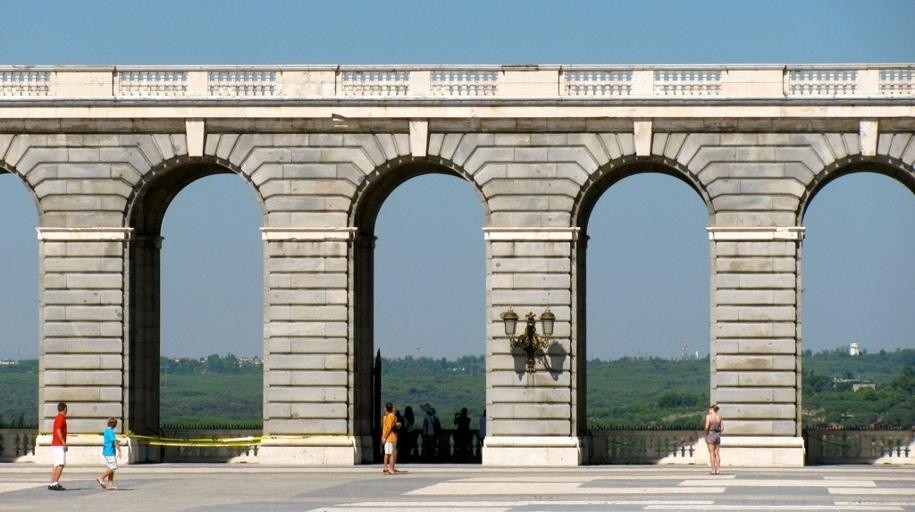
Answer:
[421,403,433,413]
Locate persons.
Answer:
[98,417,121,490]
[382,403,487,474]
[705,405,723,475]
[47,403,68,490]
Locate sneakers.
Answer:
[97,478,106,489]
[52,483,65,491]
